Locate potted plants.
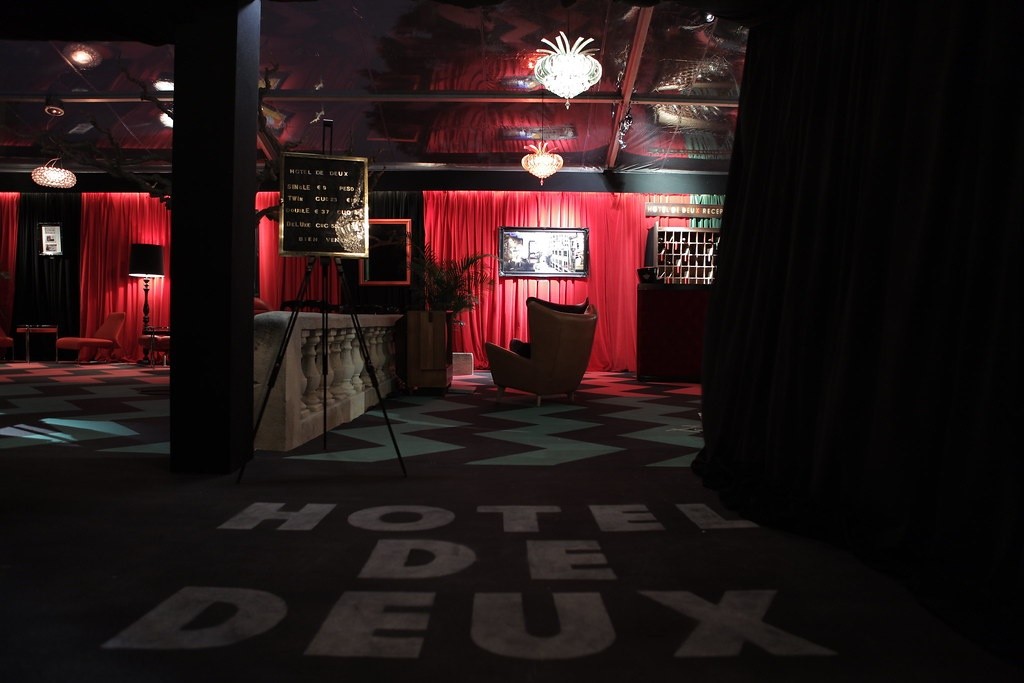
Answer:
[405,233,505,366]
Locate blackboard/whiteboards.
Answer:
[359,218,411,285]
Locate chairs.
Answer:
[485,303,597,407]
[510,297,589,359]
[56,313,125,363]
[0,327,14,362]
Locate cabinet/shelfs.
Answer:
[407,311,453,397]
[646,221,721,284]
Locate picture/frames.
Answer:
[358,219,411,286]
[498,226,590,278]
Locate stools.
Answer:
[139,335,170,367]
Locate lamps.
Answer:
[533,0,604,110]
[128,244,164,365]
[521,85,564,187]
[31,153,78,189]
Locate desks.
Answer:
[16,328,59,363]
